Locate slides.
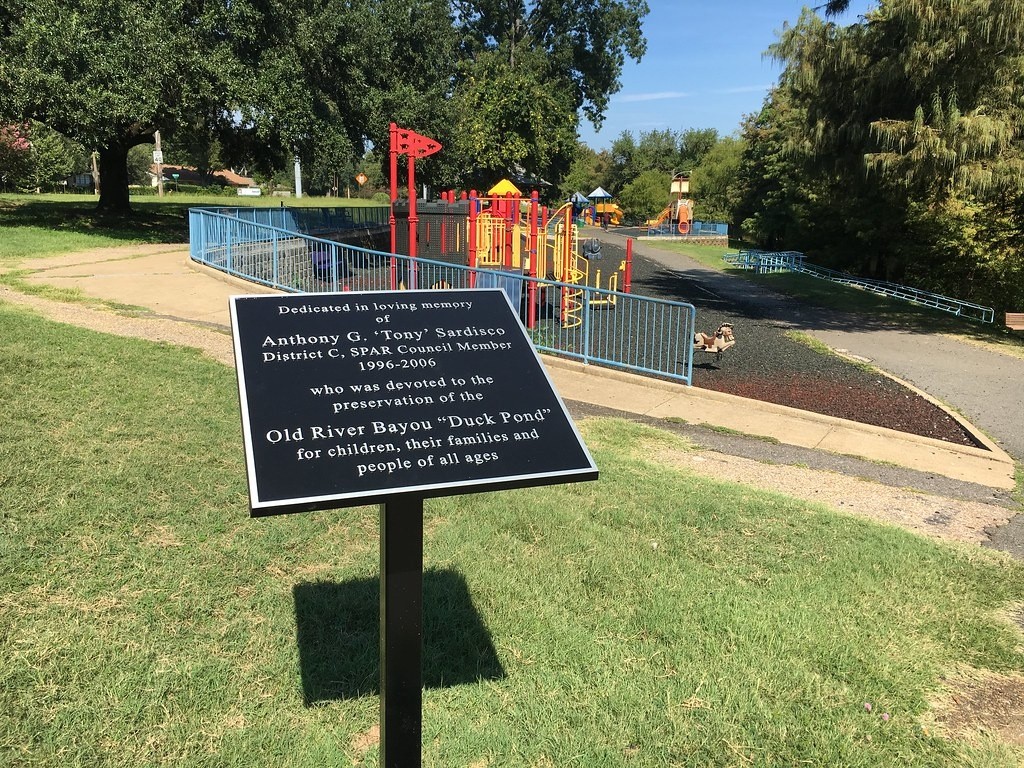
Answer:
[678,206,689,233]
[611,208,623,224]
[475,269,522,318]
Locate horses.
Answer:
[693,323,735,361]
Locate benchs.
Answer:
[1004,312,1024,331]
[310,239,352,280]
